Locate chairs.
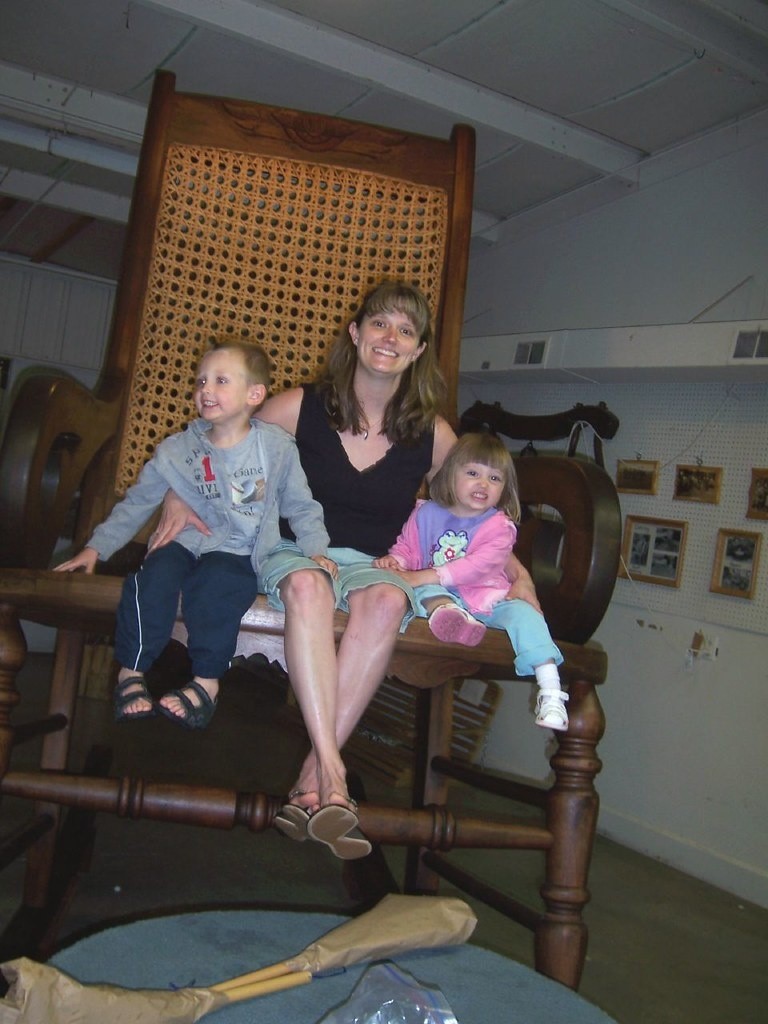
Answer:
[0,68,622,992]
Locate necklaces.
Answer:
[361,419,383,440]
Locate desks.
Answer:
[0,909,620,1024]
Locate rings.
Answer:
[154,532,160,538]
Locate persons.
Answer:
[676,470,714,498]
[752,478,768,512]
[632,534,648,565]
[52,341,339,734]
[144,282,544,860]
[372,432,570,731]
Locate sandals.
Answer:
[534,688,570,731]
[112,676,156,723]
[158,681,218,728]
[428,604,486,646]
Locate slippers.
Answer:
[273,789,311,843]
[307,796,371,859]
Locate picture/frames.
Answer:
[617,514,688,588]
[745,468,768,520]
[673,464,723,504]
[615,459,659,495]
[709,528,762,600]
